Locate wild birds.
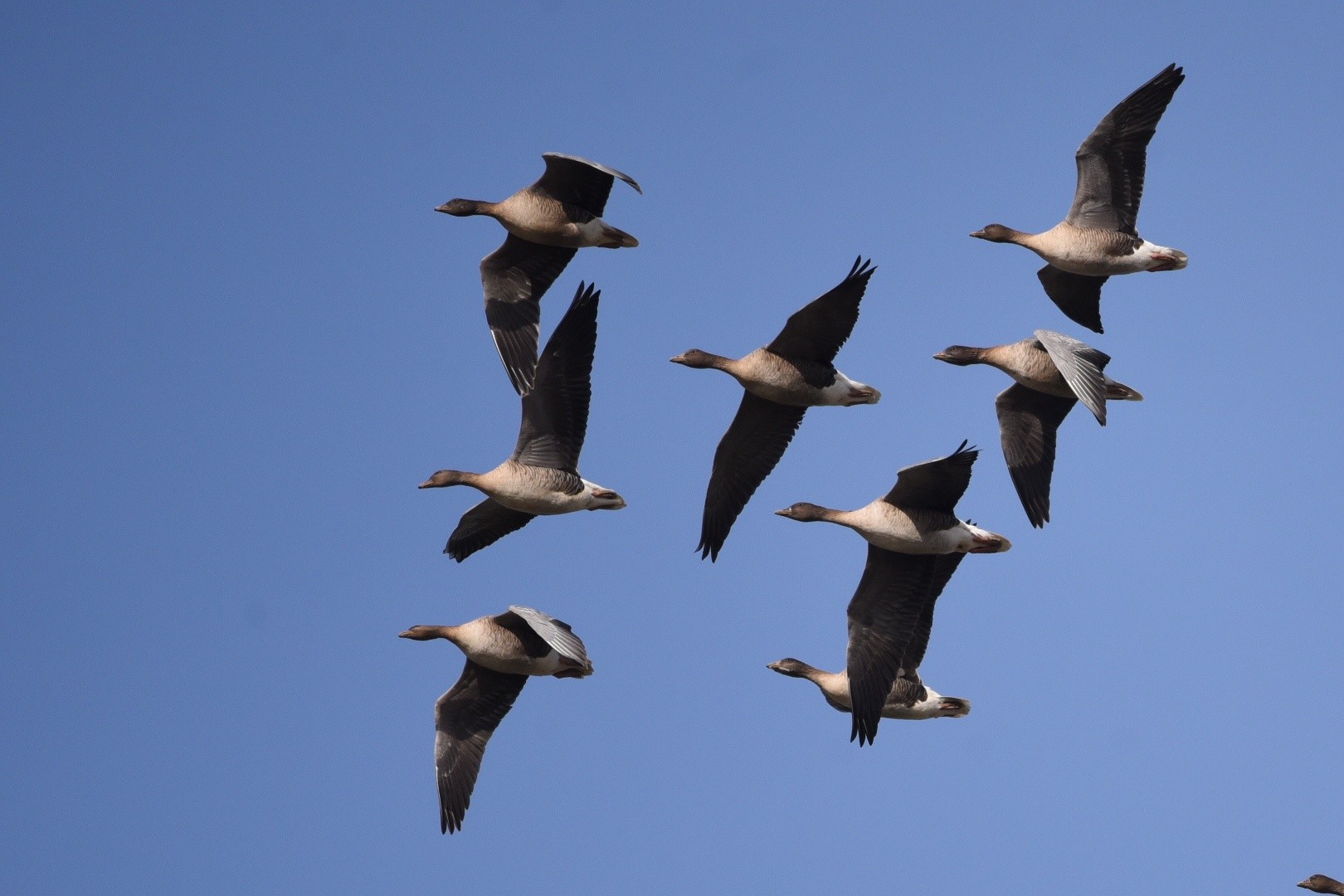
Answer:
[434,152,643,398]
[417,280,630,565]
[773,438,1014,749]
[968,61,1191,334]
[397,603,595,836]
[666,254,884,563]
[1296,875,1344,895]
[932,330,1142,529]
